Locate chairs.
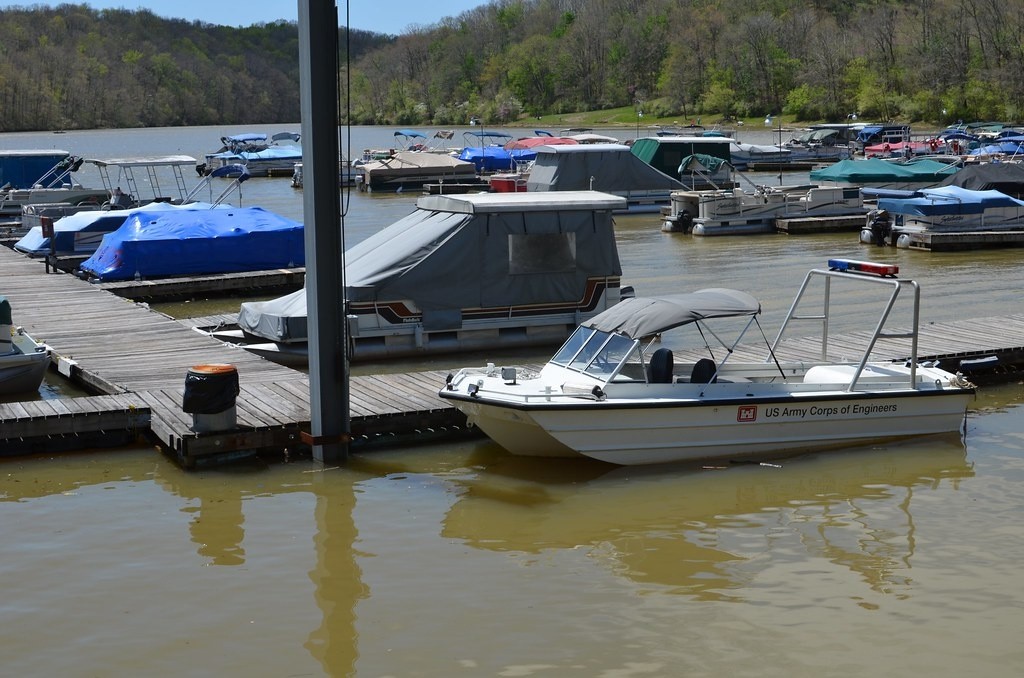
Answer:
[690,358,717,383]
[646,348,674,383]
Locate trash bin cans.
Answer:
[186,361,239,431]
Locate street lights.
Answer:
[764,114,783,186]
[636,110,644,140]
[940,109,949,155]
[847,114,858,159]
[469,117,485,176]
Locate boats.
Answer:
[656,131,792,170]
[436,257,979,467]
[859,185,1024,249]
[661,155,872,236]
[0,150,197,241]
[192,188,635,364]
[791,122,1024,162]
[53,132,66,134]
[203,132,301,175]
[293,126,620,187]
